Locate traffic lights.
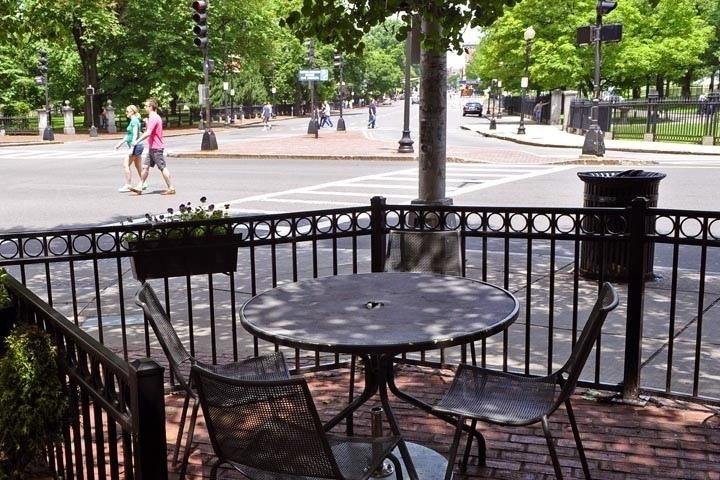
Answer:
[333,49,341,68]
[577,26,596,42]
[601,25,622,40]
[191,0,208,57]
[305,40,311,65]
[599,1,617,14]
[38,49,47,74]
[85,88,95,95]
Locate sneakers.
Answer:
[118,182,175,195]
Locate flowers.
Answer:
[122,196,232,237]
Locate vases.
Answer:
[125,234,245,278]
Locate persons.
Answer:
[533,100,550,125]
[101,106,109,129]
[128,97,176,195]
[115,104,147,192]
[261,97,376,132]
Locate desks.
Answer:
[240,271,521,479]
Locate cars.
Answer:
[411,97,420,104]
[382,98,391,105]
[463,102,483,118]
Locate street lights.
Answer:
[497,81,503,119]
[524,25,536,70]
[272,88,277,116]
[517,77,528,134]
[229,89,236,124]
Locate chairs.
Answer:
[189,364,403,479]
[430,280,618,478]
[135,280,295,479]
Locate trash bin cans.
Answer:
[577,170,667,283]
